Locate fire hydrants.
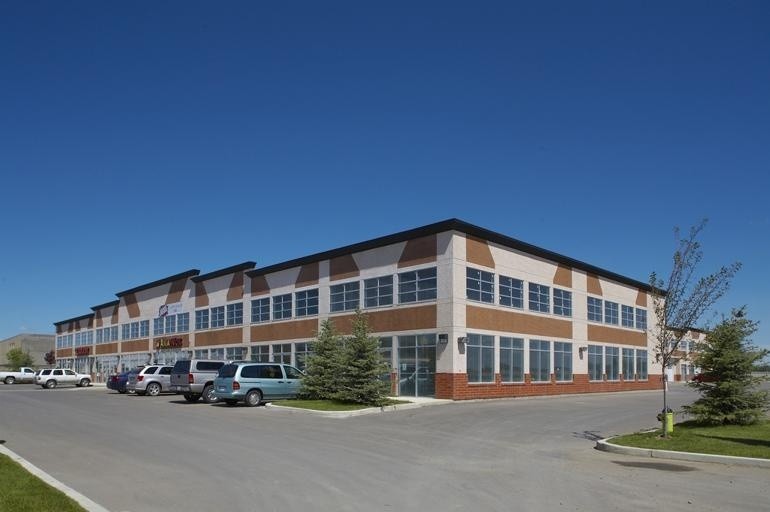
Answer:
[656,405,675,434]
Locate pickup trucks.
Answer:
[0,366,38,384]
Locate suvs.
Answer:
[106,358,312,405]
[378,362,435,398]
[35,368,92,388]
